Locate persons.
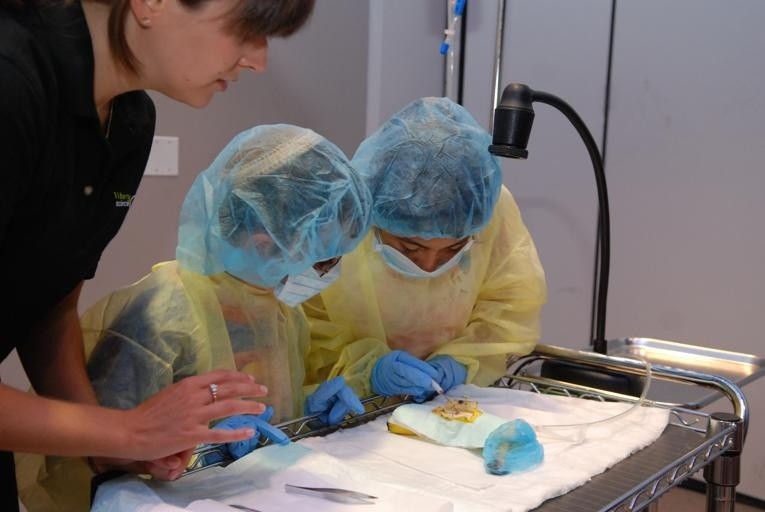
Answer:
[12,123,373,511]
[0,1,315,512]
[302,95,548,435]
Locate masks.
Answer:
[274,243,341,309]
[371,228,478,280]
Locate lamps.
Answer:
[484,81,652,398]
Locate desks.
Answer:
[89,338,749,512]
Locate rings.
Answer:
[209,384,218,401]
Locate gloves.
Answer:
[202,405,291,465]
[302,375,365,428]
[413,353,469,404]
[371,349,439,399]
